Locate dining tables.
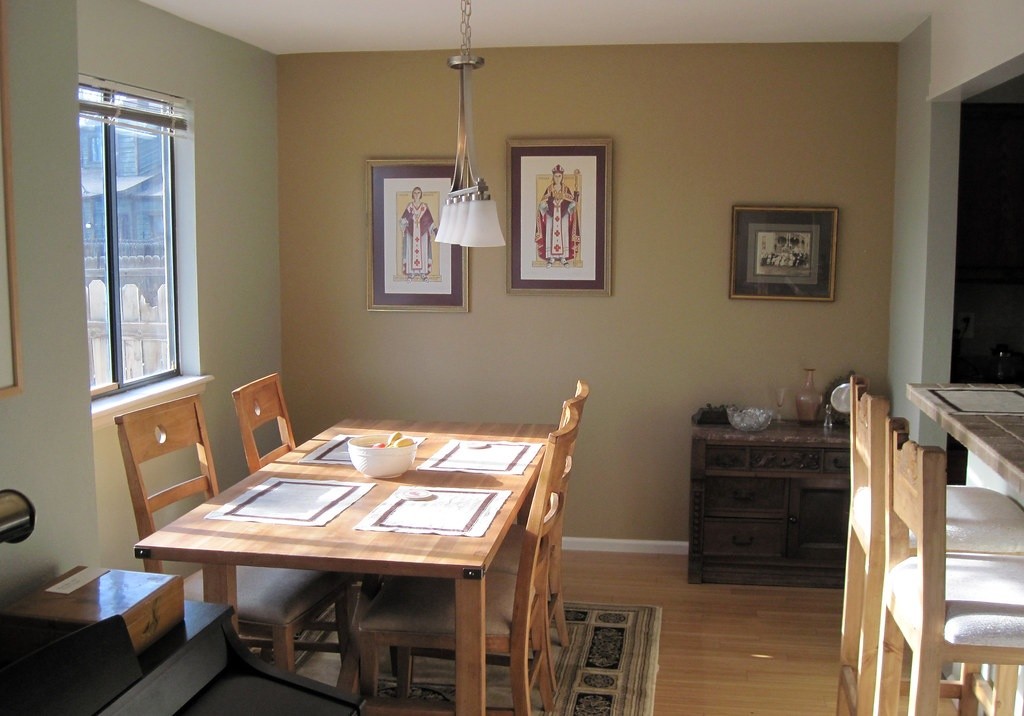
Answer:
[912,373,1024,502]
[132,411,563,716]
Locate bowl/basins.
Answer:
[727,407,773,431]
[347,434,417,479]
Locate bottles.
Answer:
[824,404,832,427]
[796,368,823,427]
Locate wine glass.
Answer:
[775,390,785,424]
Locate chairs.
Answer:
[114,393,354,687]
[869,427,1024,716]
[495,380,590,660]
[354,410,583,714]
[232,372,299,474]
[830,375,983,716]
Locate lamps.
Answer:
[435,1,511,249]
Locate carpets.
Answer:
[237,589,663,715]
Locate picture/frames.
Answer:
[504,134,614,298]
[727,204,842,304]
[364,156,472,314]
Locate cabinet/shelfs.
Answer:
[685,409,851,591]
